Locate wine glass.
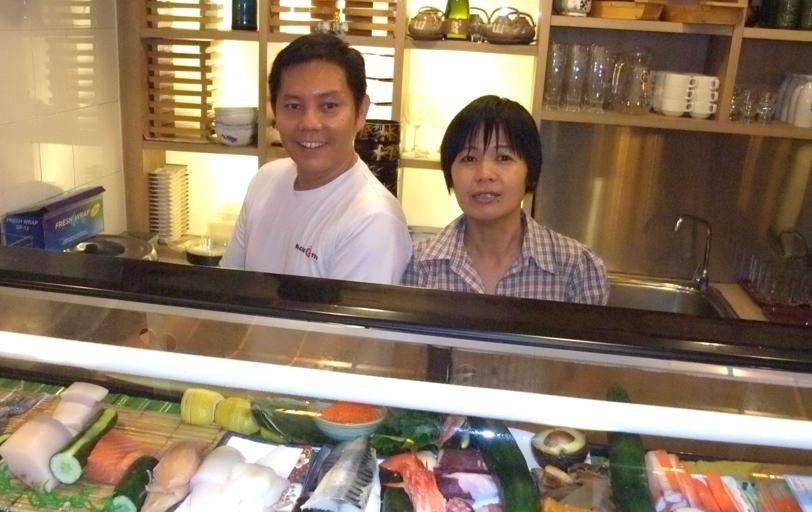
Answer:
[729,87,779,125]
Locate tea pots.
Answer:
[410,8,444,40]
[469,7,535,46]
[617,42,655,113]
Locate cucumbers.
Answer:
[111,455,158,512]
[50,407,118,484]
[465,415,540,512]
[382,486,412,512]
[605,382,654,512]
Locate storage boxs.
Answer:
[5,186,107,251]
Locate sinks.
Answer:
[605,274,740,319]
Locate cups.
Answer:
[542,41,614,117]
[653,69,720,122]
[232,1,258,29]
[738,244,811,304]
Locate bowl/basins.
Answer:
[308,399,389,441]
[214,105,258,147]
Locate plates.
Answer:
[184,238,228,256]
[147,162,191,243]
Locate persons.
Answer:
[401,96,610,306]
[216,27,412,286]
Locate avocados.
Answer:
[530,428,588,473]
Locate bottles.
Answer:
[778,73,812,129]
[444,0,470,41]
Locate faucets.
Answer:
[672,212,711,290]
